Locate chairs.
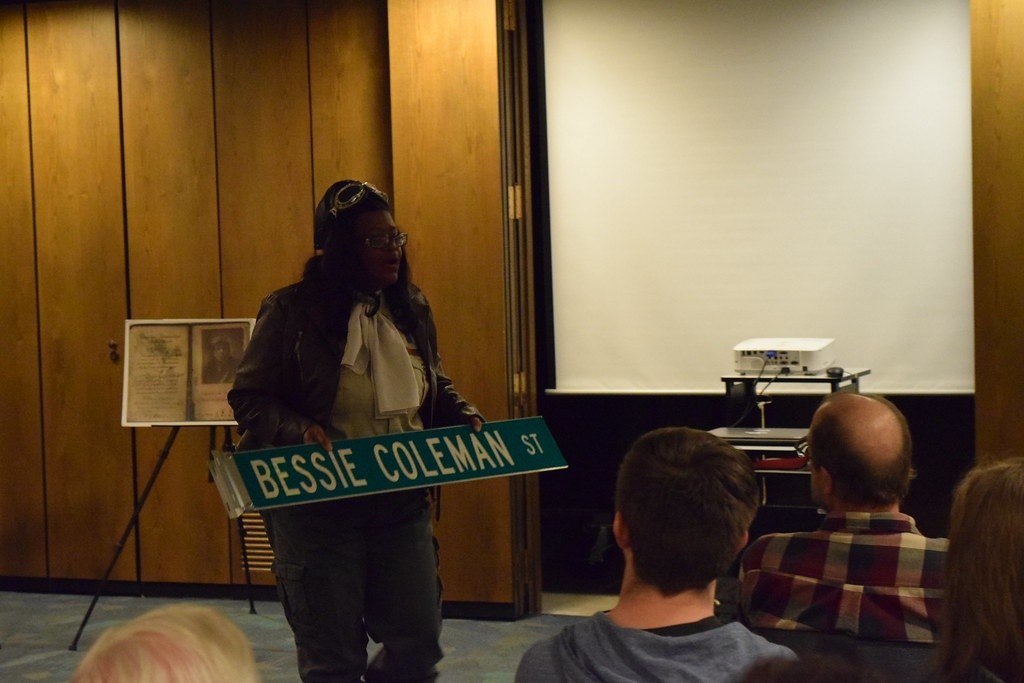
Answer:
[749,627,941,682]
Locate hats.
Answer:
[314,180,389,249]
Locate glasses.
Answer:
[328,181,389,211]
[794,435,836,481]
[347,233,408,250]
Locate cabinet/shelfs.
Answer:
[717,369,873,507]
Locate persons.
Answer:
[70,603,260,683]
[738,391,951,642]
[227,181,487,682]
[513,426,797,683]
[930,457,1024,683]
[202,333,240,384]
